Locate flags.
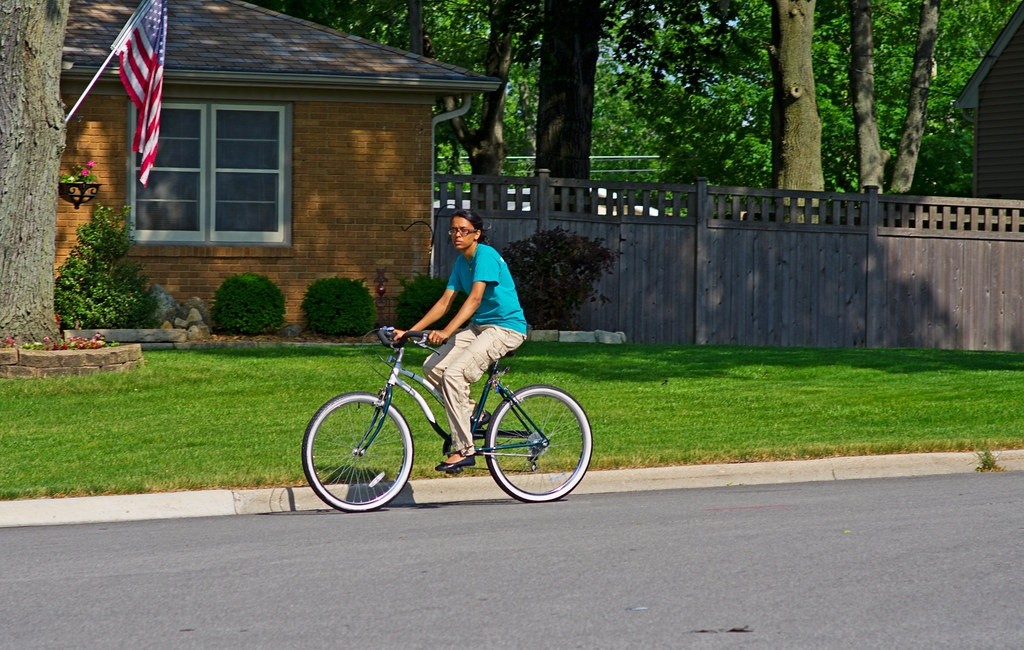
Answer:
[111,0,167,188]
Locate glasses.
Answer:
[448,229,477,236]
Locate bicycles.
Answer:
[301,326,594,515]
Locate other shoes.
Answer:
[472,410,491,429]
[435,455,475,471]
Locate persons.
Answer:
[392,209,528,471]
